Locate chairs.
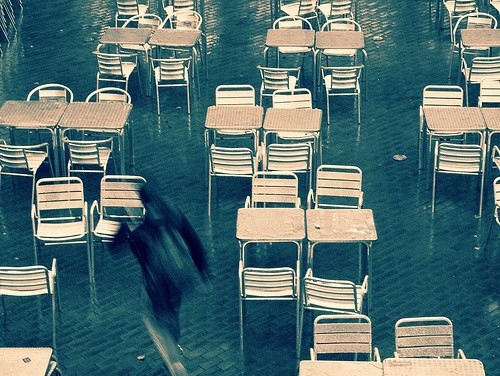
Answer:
[0,0,384,364]
[391,0,500,360]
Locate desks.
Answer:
[96,28,151,100]
[457,28,500,75]
[263,108,324,168]
[0,347,58,376]
[479,108,500,157]
[148,28,208,99]
[300,359,383,376]
[58,102,133,181]
[261,29,316,85]
[422,106,486,191]
[236,207,305,277]
[204,104,263,177]
[315,30,367,96]
[305,208,379,275]
[0,97,68,175]
[385,358,484,376]
